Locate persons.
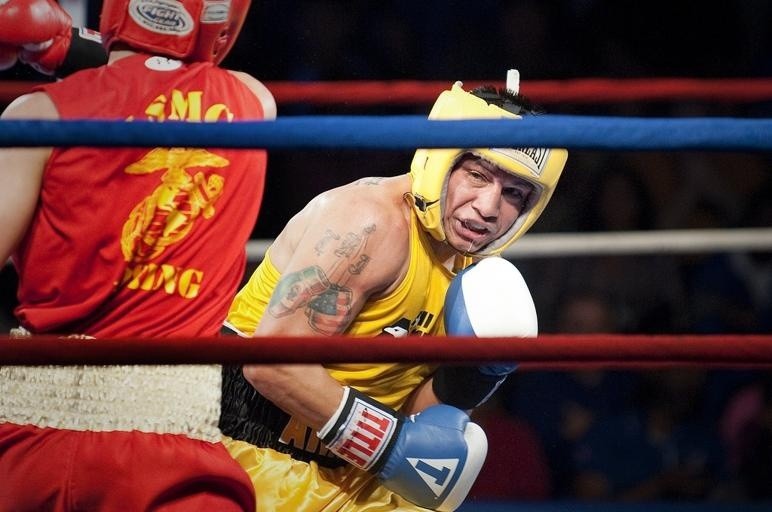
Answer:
[0,1,279,510]
[219,77,571,510]
[466,149,772,506]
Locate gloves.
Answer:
[431,255,538,411]
[0,1,107,79]
[318,381,490,512]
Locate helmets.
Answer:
[100,1,253,67]
[411,81,569,259]
